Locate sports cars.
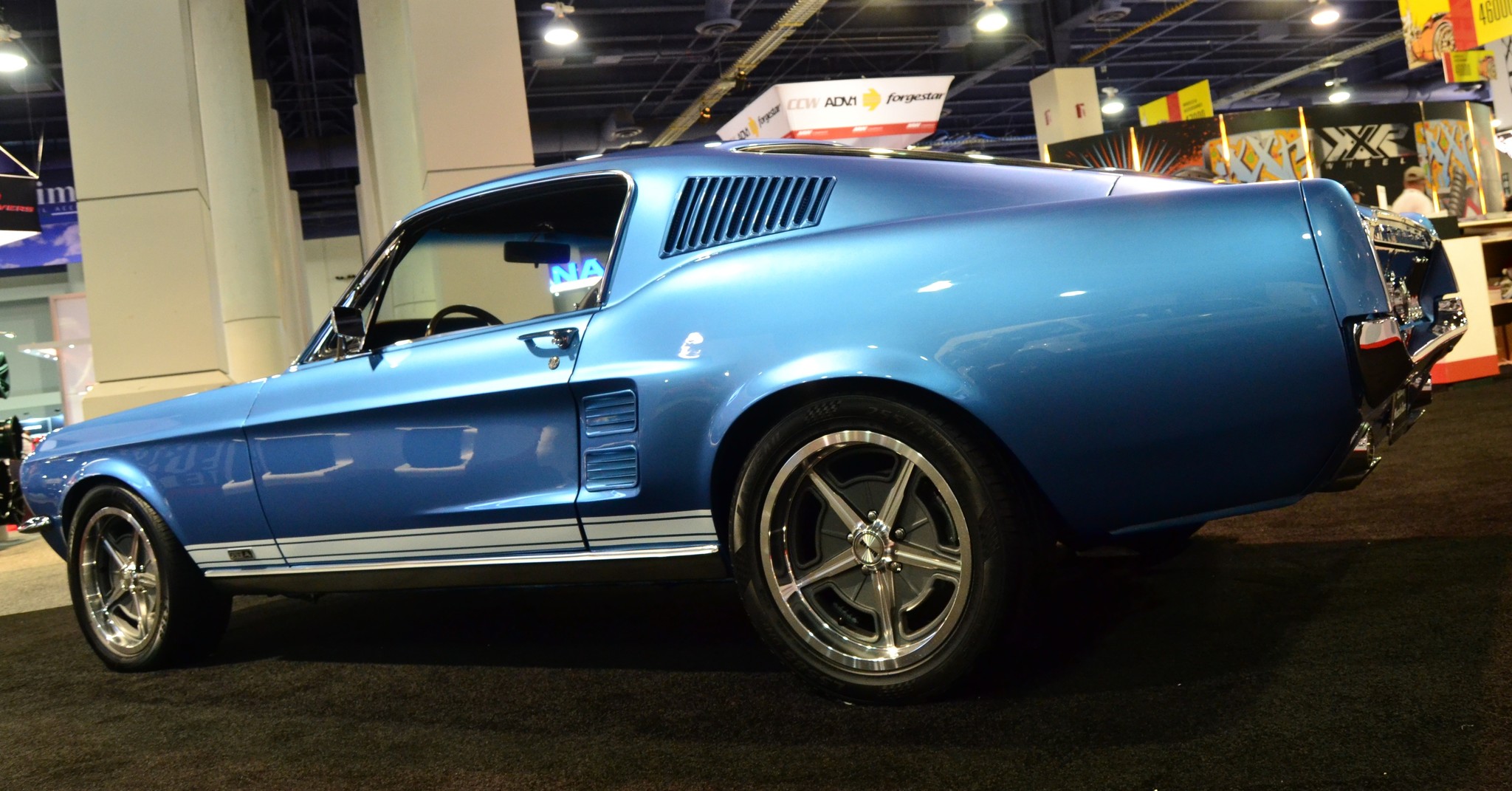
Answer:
[13,135,1469,705]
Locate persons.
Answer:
[1392,166,1436,216]
[1341,180,1366,204]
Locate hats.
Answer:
[1404,165,1427,183]
[1344,180,1366,196]
